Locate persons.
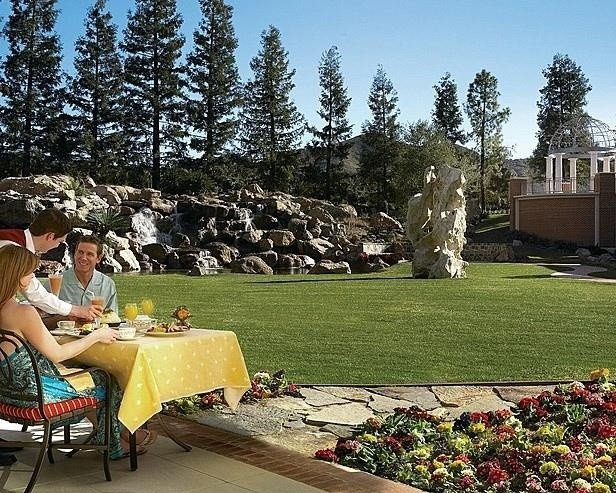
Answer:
[0,207,71,256]
[1,244,158,459]
[1,438,25,466]
[41,235,119,322]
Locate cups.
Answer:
[57,320,76,329]
[112,315,158,341]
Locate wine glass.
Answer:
[49,275,63,298]
[141,299,154,317]
[124,304,138,326]
[92,297,105,332]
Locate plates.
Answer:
[140,324,190,338]
[51,327,80,336]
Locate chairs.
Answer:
[0,329,113,493]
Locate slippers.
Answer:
[109,426,157,461]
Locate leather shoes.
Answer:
[0,453,18,467]
[0,438,25,453]
